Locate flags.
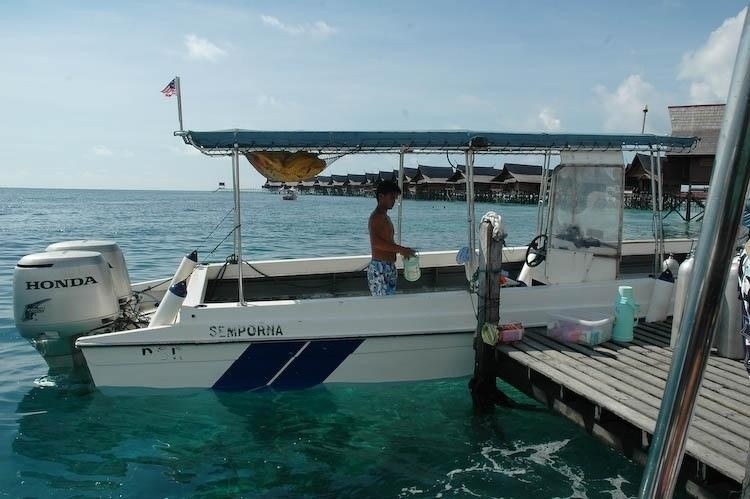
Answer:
[159,78,176,97]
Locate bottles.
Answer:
[610,284,641,343]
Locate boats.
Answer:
[283,188,297,200]
[12,77,748,391]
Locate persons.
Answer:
[365,180,420,297]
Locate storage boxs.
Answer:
[546,308,616,345]
